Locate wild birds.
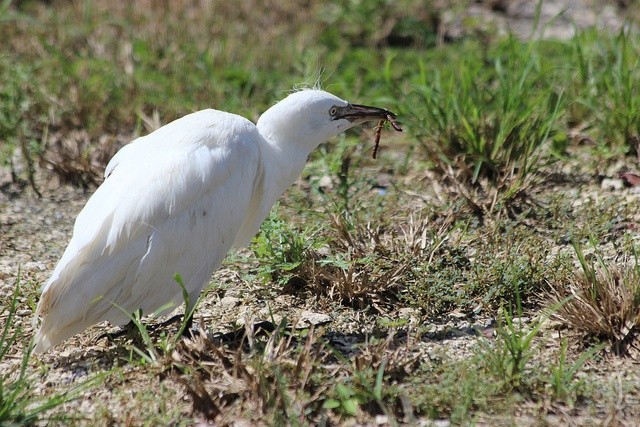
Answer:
[28,69,397,360]
